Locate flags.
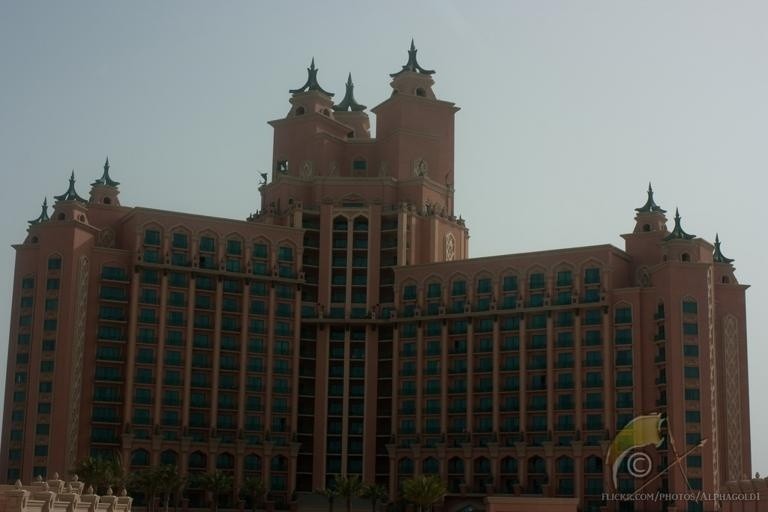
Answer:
[606,413,664,491]
